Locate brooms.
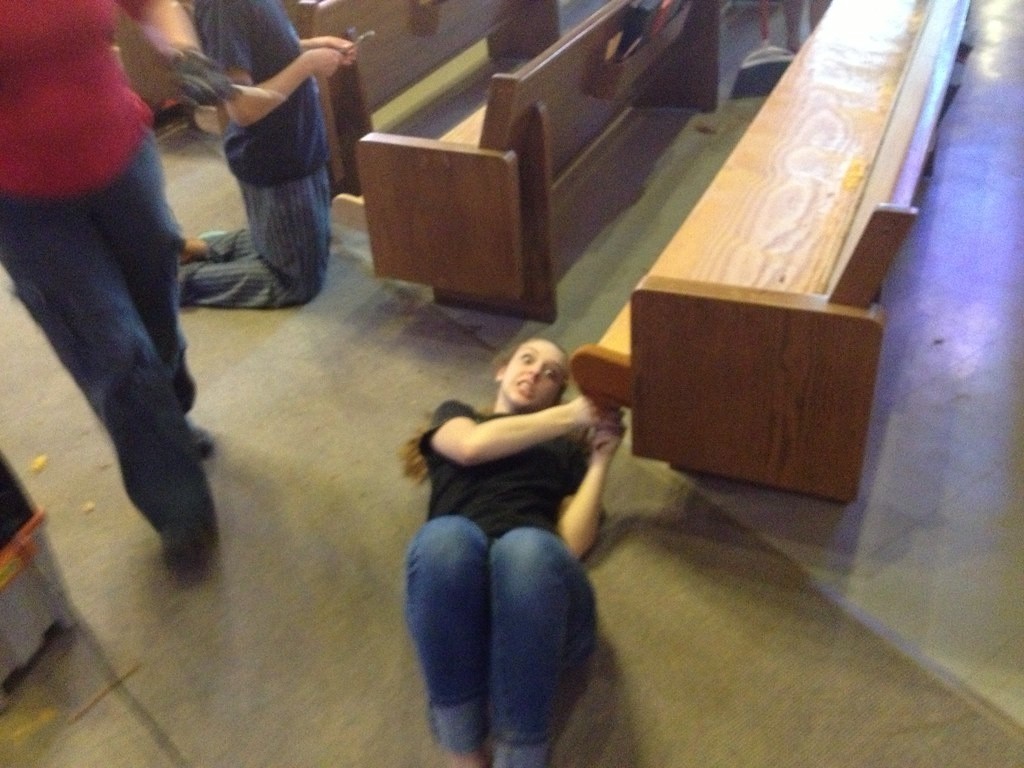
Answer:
[730,1,795,98]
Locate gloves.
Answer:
[172,50,241,107]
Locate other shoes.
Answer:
[186,412,214,459]
[160,499,215,575]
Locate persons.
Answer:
[402,338,626,767]
[1,0,242,592]
[178,0,358,311]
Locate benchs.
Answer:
[110,0,972,500]
